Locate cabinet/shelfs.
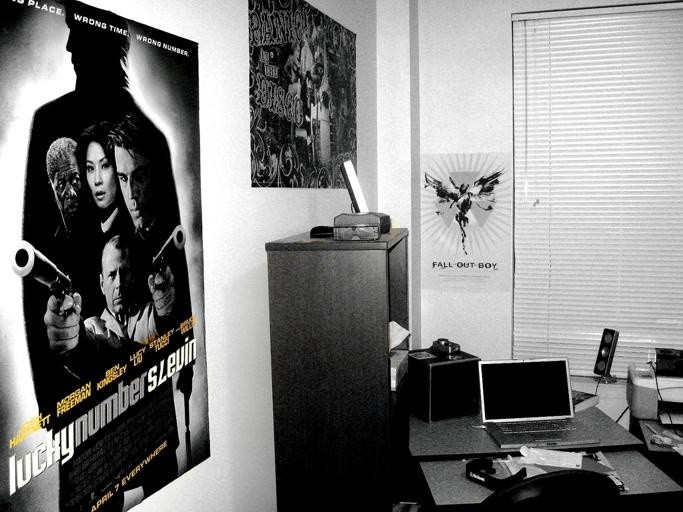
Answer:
[266,228,409,511]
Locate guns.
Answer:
[151,225,186,277]
[12,240,74,316]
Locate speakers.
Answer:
[594,328,619,377]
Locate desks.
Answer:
[408,405,683,511]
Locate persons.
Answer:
[6,3,197,512]
[105,113,159,238]
[39,234,179,358]
[66,120,125,235]
[39,133,90,241]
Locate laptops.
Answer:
[478,357,600,449]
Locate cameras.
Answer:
[433,338,460,354]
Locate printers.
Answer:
[626,348,683,425]
